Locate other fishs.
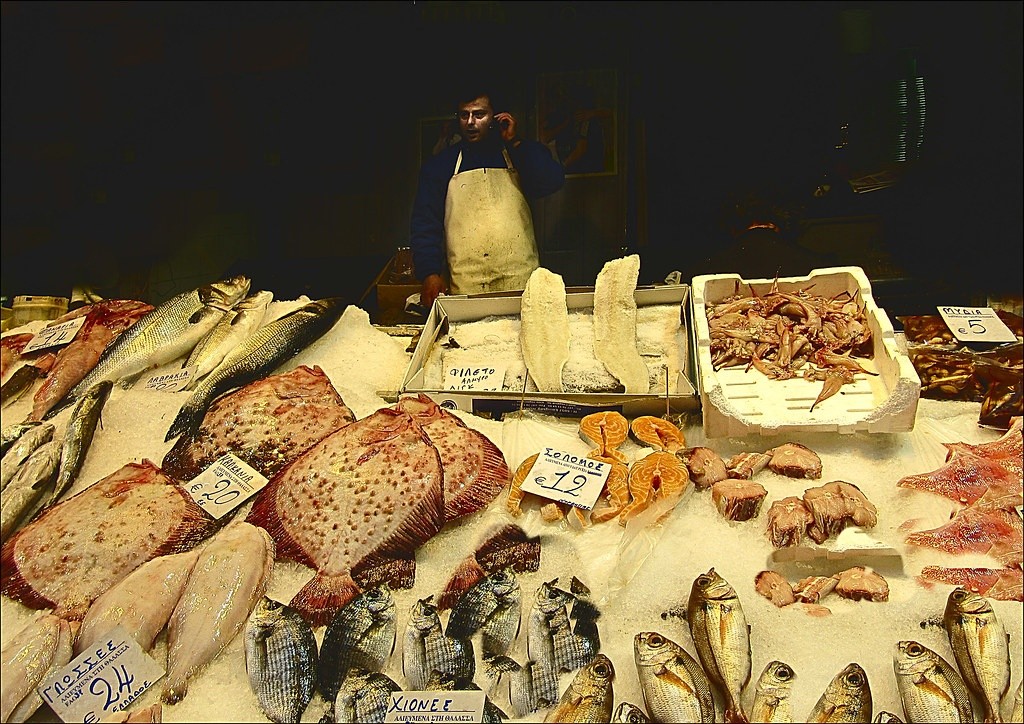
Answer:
[0,254,1024,723]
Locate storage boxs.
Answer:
[691,266,921,439]
[397,284,700,421]
[358,250,431,326]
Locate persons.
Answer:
[409,82,566,312]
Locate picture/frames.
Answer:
[536,66,618,179]
[416,116,458,170]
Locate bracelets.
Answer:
[507,135,519,147]
